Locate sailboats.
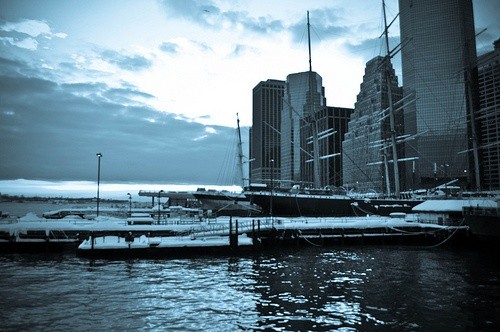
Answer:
[0,8,470,255]
